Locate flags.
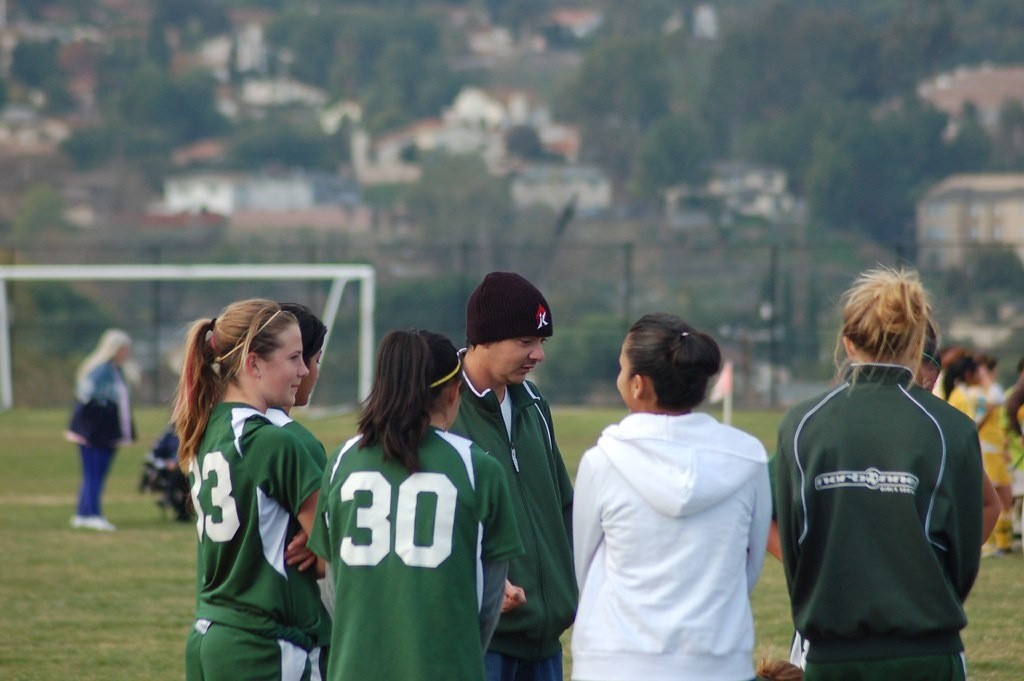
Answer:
[709,363,727,403]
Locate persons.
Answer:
[446,272,579,681]
[306,330,525,681]
[755,267,1024,681]
[63,327,137,531]
[173,299,332,681]
[570,313,772,681]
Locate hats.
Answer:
[466,272,553,344]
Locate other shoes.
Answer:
[68,515,116,532]
